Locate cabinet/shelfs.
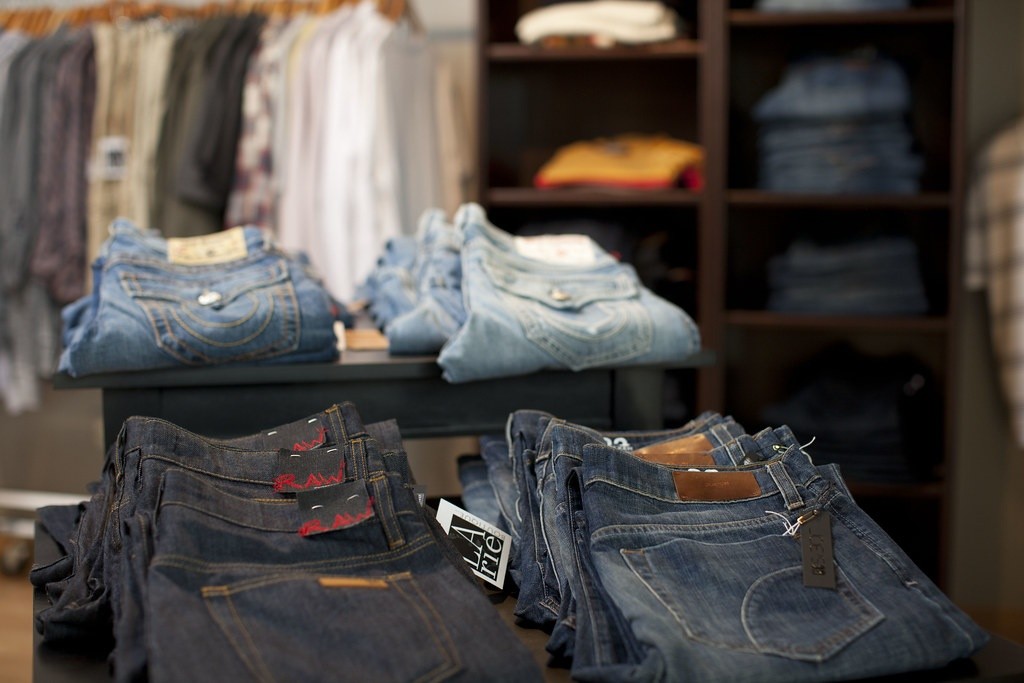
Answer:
[476,1,980,605]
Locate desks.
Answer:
[53,349,716,457]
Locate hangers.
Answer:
[1,1,434,34]
